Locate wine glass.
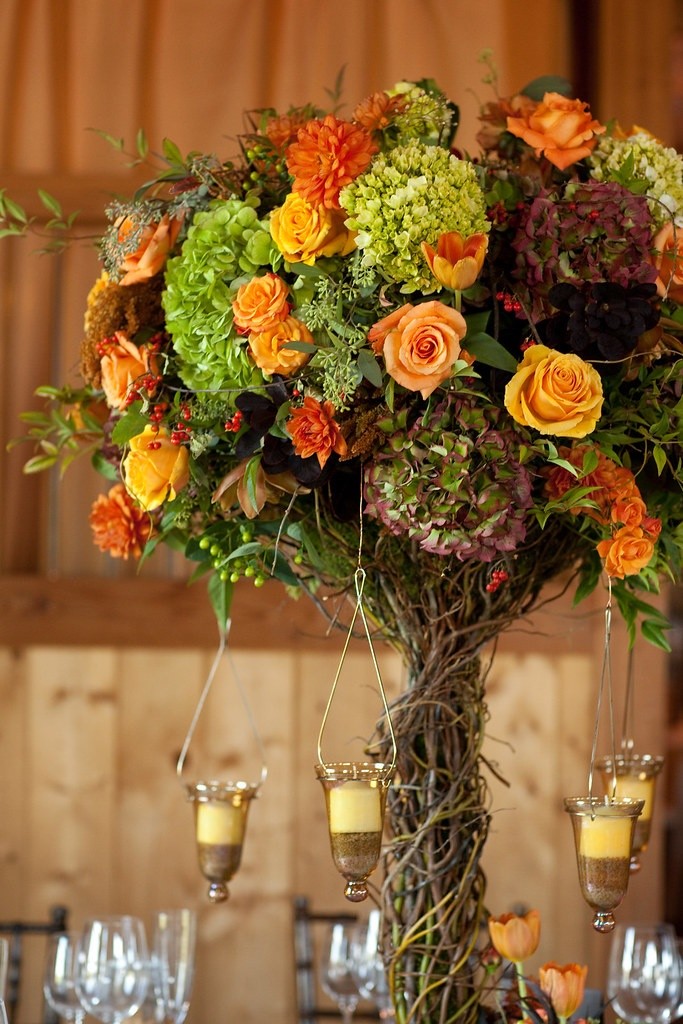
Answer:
[318,921,362,1024]
[350,906,418,1024]
[155,908,195,1024]
[42,930,89,1024]
[74,915,150,1024]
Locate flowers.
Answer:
[0,49,683,1024]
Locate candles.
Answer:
[579,795,633,858]
[606,761,654,819]
[197,803,243,845]
[330,765,382,833]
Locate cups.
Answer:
[606,922,683,1024]
